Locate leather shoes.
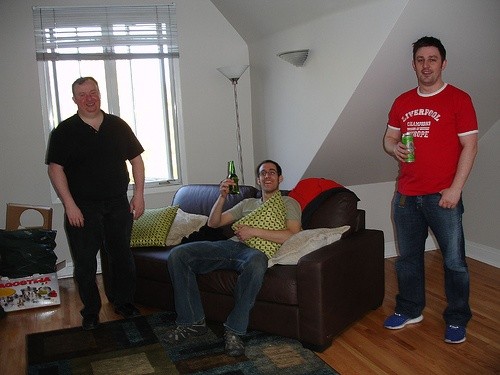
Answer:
[115,304,141,318]
[82,316,98,330]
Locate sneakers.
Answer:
[445,320,466,343]
[383,310,424,329]
[223,328,245,356]
[165,321,208,344]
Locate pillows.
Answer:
[166,208,209,246]
[232,191,288,259]
[268,226,350,267]
[129,205,179,247]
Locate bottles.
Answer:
[227,161,239,194]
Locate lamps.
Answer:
[217,64,258,185]
[276,49,309,67]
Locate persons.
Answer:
[161,160,302,355]
[45,76,145,328]
[383,36,479,343]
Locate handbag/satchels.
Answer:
[0,229,58,279]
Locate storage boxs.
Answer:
[0,259,66,312]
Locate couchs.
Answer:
[125,178,386,353]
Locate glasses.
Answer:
[262,172,277,175]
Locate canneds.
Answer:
[402,134,415,162]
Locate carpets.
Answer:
[26,311,340,375]
